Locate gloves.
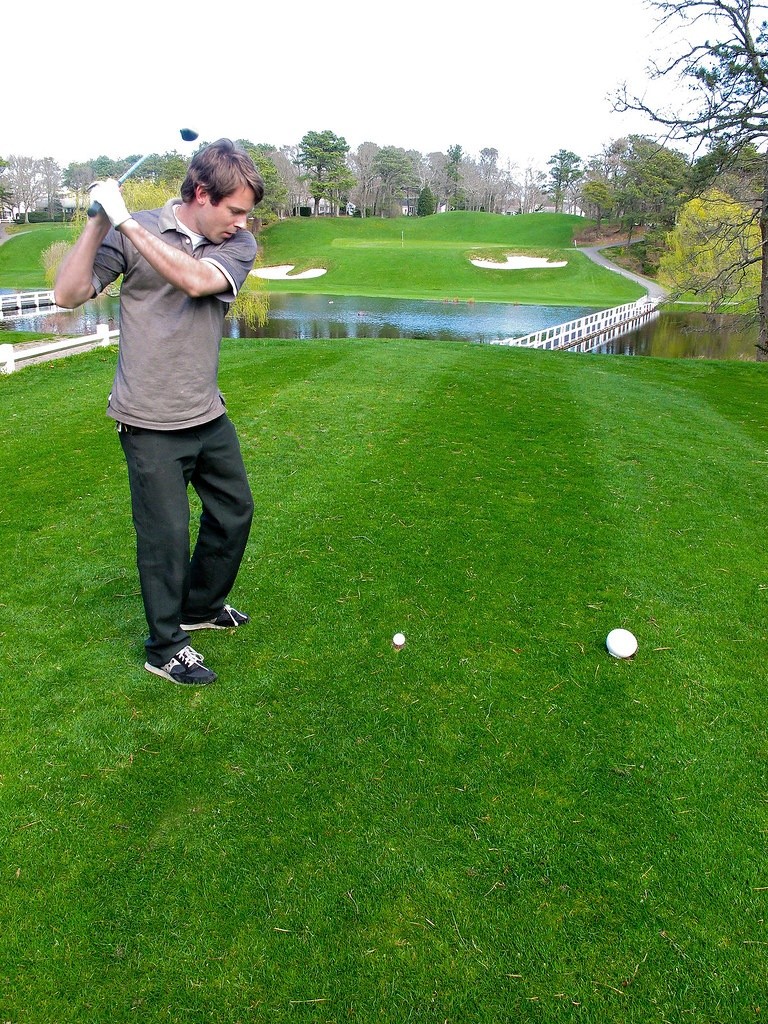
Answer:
[91,178,132,230]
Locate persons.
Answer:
[53,137,263,689]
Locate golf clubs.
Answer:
[83,128,201,217]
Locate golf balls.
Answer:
[392,632,406,647]
[606,628,639,660]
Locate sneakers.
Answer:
[143,649,218,687]
[180,605,248,631]
